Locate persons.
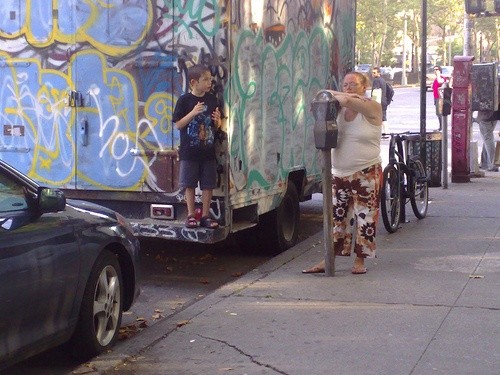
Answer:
[432,66,446,131]
[346,63,352,72]
[477,76,500,172]
[379,65,392,74]
[371,67,388,139]
[172,65,221,229]
[302,72,382,275]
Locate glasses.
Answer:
[434,69,438,71]
[372,72,377,74]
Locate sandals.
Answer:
[185,215,197,228]
[200,216,219,228]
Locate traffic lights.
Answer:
[464,0,486,15]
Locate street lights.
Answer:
[395,8,415,85]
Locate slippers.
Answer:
[302,265,325,274]
[352,264,368,274]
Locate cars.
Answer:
[355,63,402,77]
[425,66,453,92]
[0,159,142,375]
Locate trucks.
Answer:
[0,0,357,253]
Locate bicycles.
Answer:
[379,131,429,233]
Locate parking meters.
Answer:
[437,83,453,188]
[310,90,341,277]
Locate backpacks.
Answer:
[386,82,395,106]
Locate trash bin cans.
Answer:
[406,132,441,186]
